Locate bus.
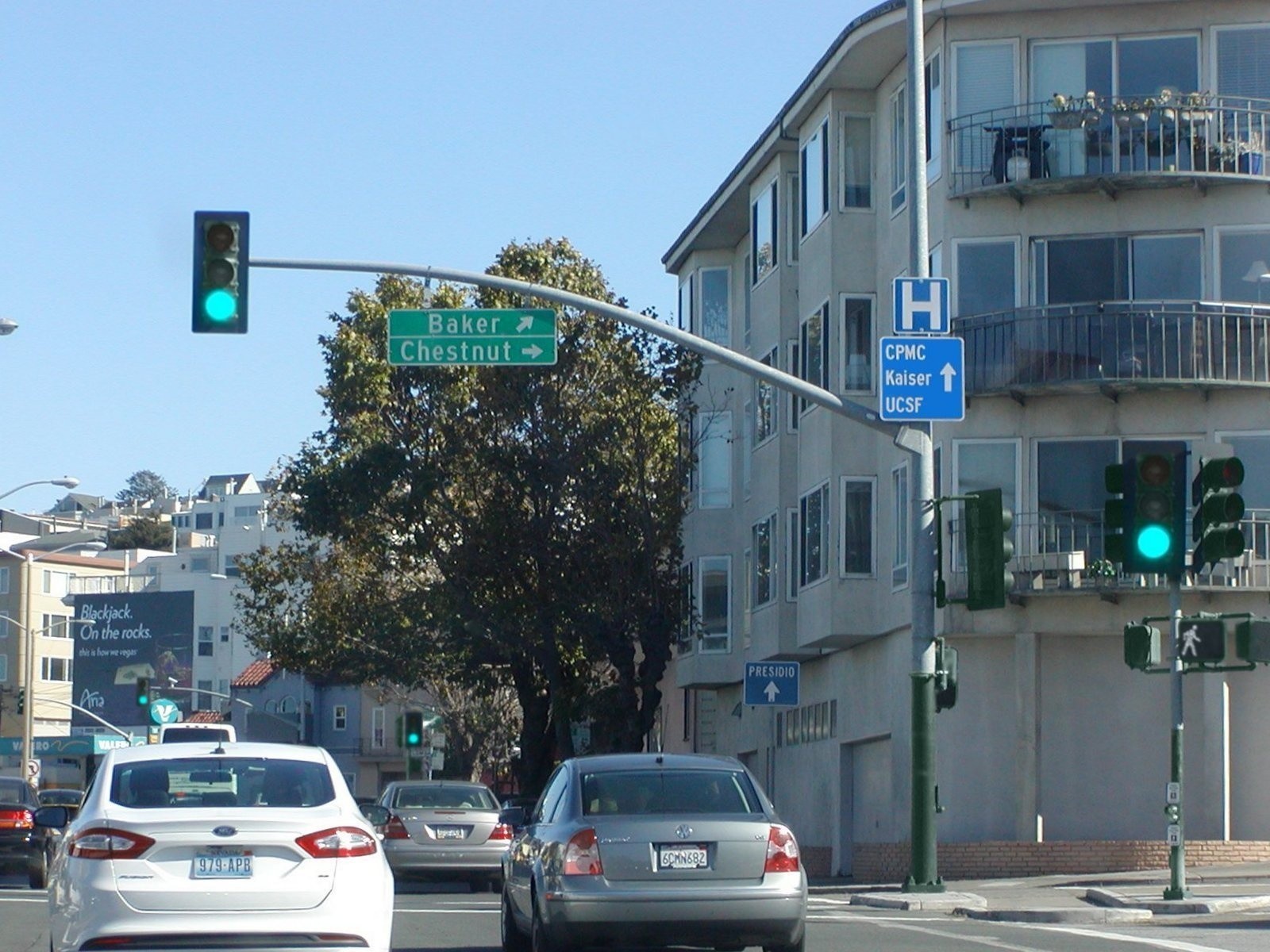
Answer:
[155,722,239,798]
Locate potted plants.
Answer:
[1188,134,1222,172]
[1048,89,1212,128]
[1211,130,1264,175]
[1088,558,1116,586]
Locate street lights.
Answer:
[0,540,107,782]
[1,608,97,755]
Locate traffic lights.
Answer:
[1234,616,1270,666]
[964,486,1015,612]
[1121,439,1188,575]
[1123,620,1162,671]
[191,209,251,335]
[1178,618,1227,663]
[1102,462,1124,563]
[405,711,424,748]
[133,677,151,708]
[1198,454,1246,563]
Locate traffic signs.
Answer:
[878,336,966,423]
[742,660,802,708]
[386,308,559,368]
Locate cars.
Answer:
[0,773,86,890]
[500,797,540,816]
[31,742,395,952]
[498,750,809,952]
[372,779,514,894]
